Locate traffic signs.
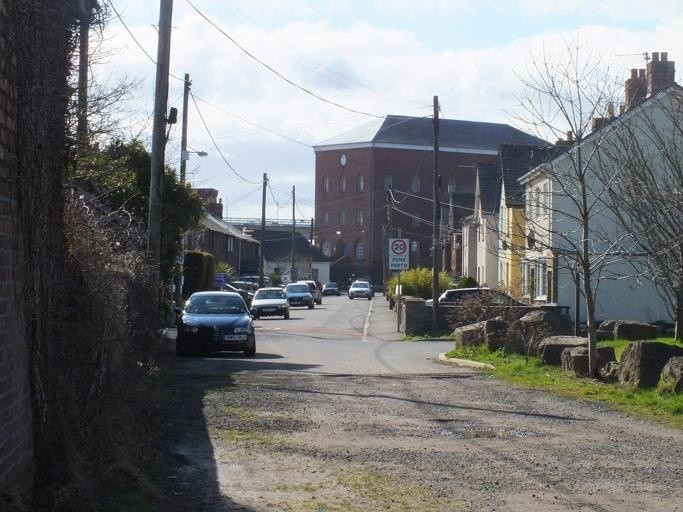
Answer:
[389,238,410,270]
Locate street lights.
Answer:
[180,151,208,184]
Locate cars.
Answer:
[230,275,322,320]
[322,282,340,296]
[438,288,531,306]
[173,291,257,357]
[348,278,374,300]
